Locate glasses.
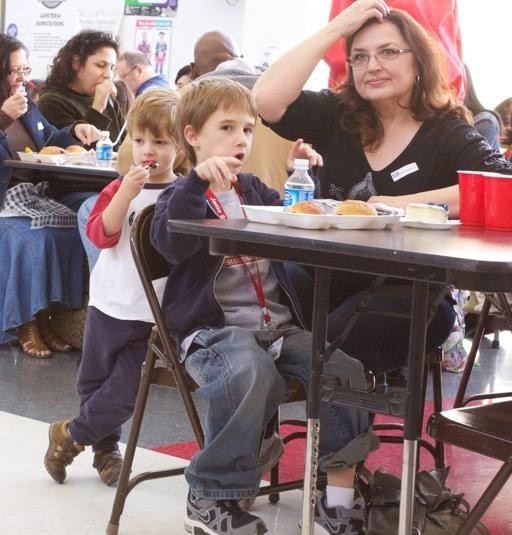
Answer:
[120,66,137,79]
[345,48,412,67]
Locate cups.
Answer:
[483,173,512,232]
[457,170,498,227]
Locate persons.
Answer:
[251,0,512,374]
[0,34,85,359]
[462,63,505,152]
[39,31,131,212]
[149,77,379,535]
[155,30,168,76]
[175,65,194,90]
[44,86,181,485]
[115,49,170,98]
[180,30,268,98]
[140,32,149,58]
[492,97,512,160]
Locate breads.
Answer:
[288,202,327,214]
[335,200,379,217]
[66,145,86,153]
[40,146,67,154]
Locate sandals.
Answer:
[15,323,52,358]
[39,316,74,352]
[7,67,33,76]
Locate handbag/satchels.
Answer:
[356,463,490,535]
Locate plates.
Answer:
[400,217,463,230]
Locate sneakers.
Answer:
[44,420,84,483]
[185,488,268,535]
[92,449,132,485]
[297,491,366,534]
[237,432,285,508]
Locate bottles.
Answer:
[95,130,114,166]
[283,159,315,205]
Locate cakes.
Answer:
[404,201,449,223]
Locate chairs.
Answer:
[426,400,512,535]
[107,203,327,530]
